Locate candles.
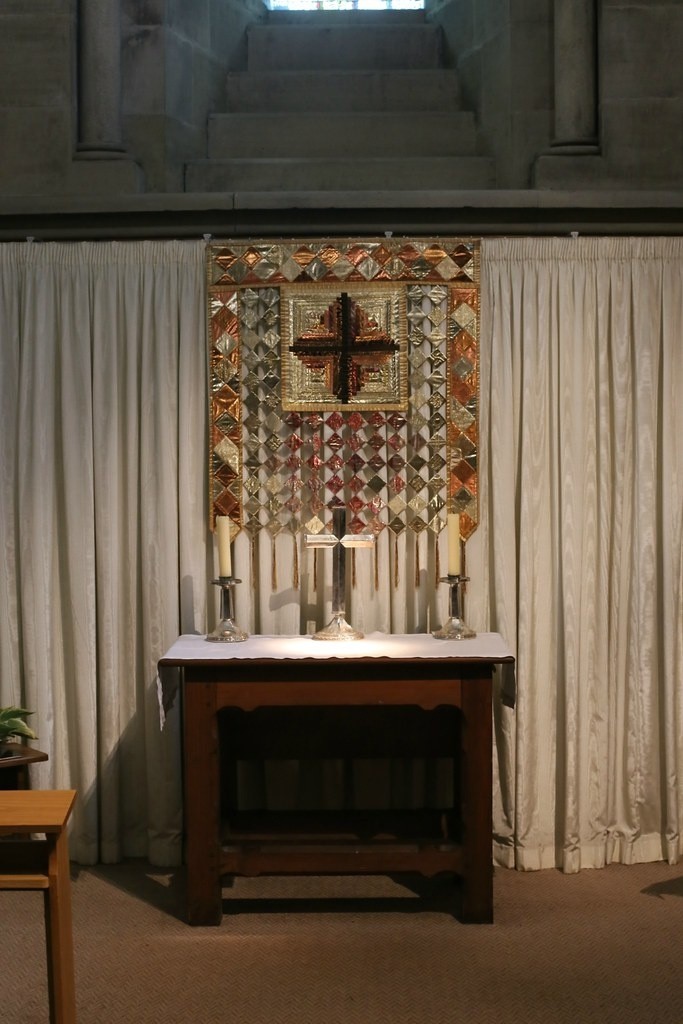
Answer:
[215,515,233,578]
[332,505,347,612]
[446,513,461,577]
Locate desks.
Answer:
[0,791,78,1024]
[155,635,518,925]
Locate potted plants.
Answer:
[0,706,48,792]
